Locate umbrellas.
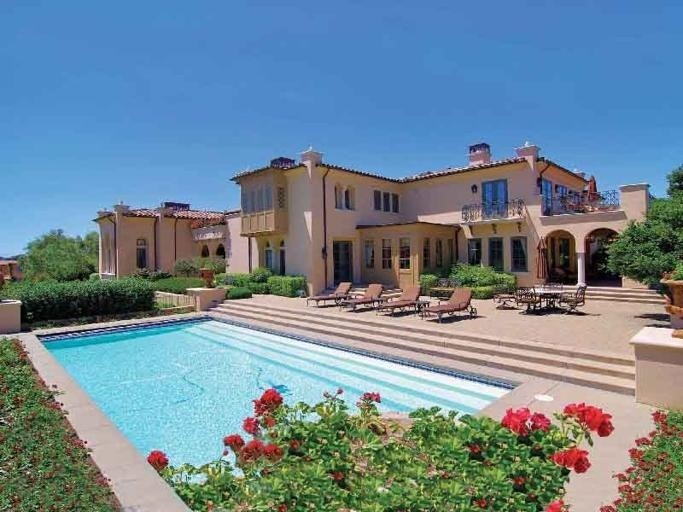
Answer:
[535,236,549,290]
[586,173,599,207]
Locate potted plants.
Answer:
[596,197,683,339]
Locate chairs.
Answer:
[305,282,477,324]
[493,281,586,314]
